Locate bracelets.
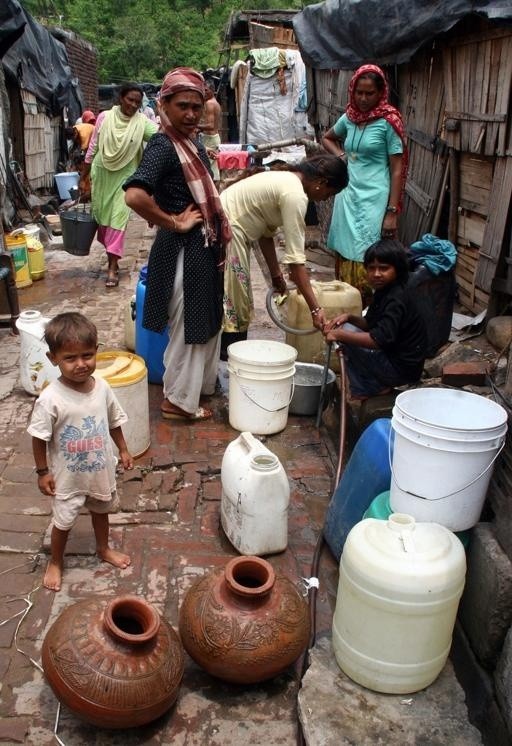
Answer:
[310,305,321,315]
[337,152,345,158]
[34,465,50,475]
[385,204,398,214]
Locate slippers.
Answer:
[106,274,119,286]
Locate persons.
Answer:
[321,236,426,401]
[69,78,223,288]
[218,152,350,365]
[25,309,134,593]
[123,66,233,424]
[321,63,412,311]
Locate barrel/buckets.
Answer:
[284,280,363,374]
[4,224,44,288]
[360,491,393,521]
[388,389,511,532]
[225,340,298,434]
[323,418,394,561]
[87,351,149,459]
[60,194,98,256]
[54,171,79,199]
[330,514,467,691]
[124,295,136,352]
[14,310,61,395]
[135,267,171,385]
[219,432,291,554]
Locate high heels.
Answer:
[161,407,212,422]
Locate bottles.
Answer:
[40,593,185,731]
[181,552,312,688]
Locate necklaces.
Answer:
[349,121,369,160]
